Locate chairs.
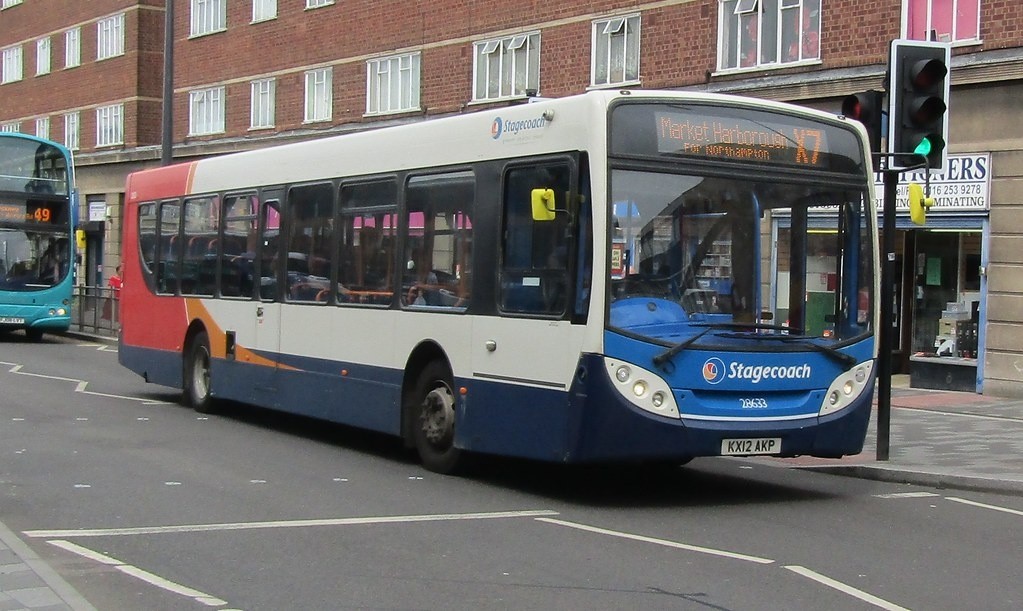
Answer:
[37,177,52,193]
[541,246,571,317]
[166,231,428,305]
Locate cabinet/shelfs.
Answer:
[685,240,732,280]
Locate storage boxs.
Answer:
[934,303,979,358]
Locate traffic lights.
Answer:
[841,90,883,171]
[888,39,951,174]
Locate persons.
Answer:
[363,251,388,286]
[405,250,439,284]
[101,264,121,325]
[545,241,591,305]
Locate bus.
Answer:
[0,131,87,342]
[125,89,935,475]
